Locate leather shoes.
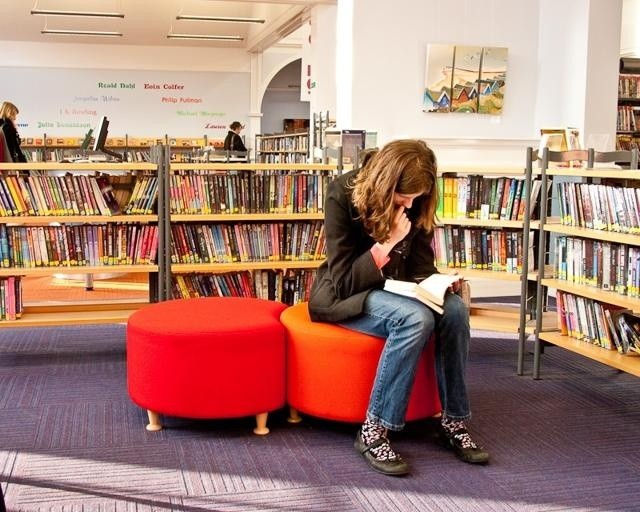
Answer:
[435,421,492,467]
[352,428,412,478]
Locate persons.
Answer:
[224,121,247,163]
[0,101,29,175]
[308,141,489,476]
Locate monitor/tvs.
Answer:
[92,116,110,152]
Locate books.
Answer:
[616,77,640,151]
[431,175,552,275]
[1,275,21,320]
[555,183,640,356]
[170,135,337,305]
[0,145,159,268]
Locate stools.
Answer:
[126,297,287,435]
[279,302,442,425]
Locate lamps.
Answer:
[41,15,123,37]
[31,1,124,19]
[166,15,244,43]
[176,0,265,23]
